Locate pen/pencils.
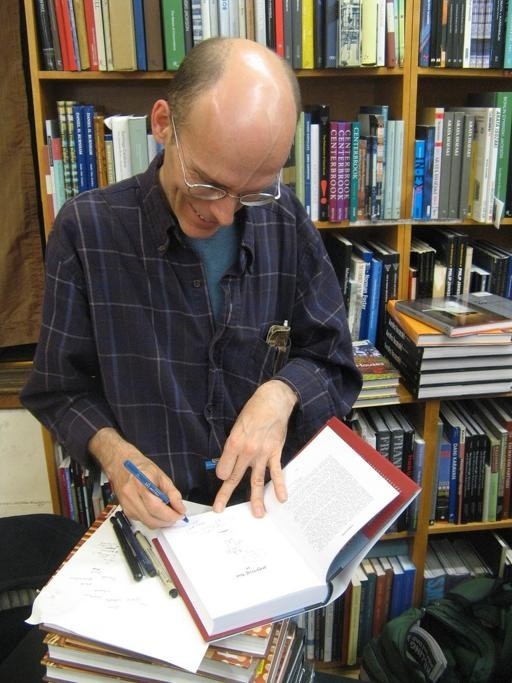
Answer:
[110,516,143,581]
[134,530,178,599]
[115,510,157,577]
[124,460,190,524]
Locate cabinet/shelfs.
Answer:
[19,0,512,683]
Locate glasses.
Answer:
[170,113,283,207]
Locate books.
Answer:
[21,1,511,683]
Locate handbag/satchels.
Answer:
[359,577,512,683]
